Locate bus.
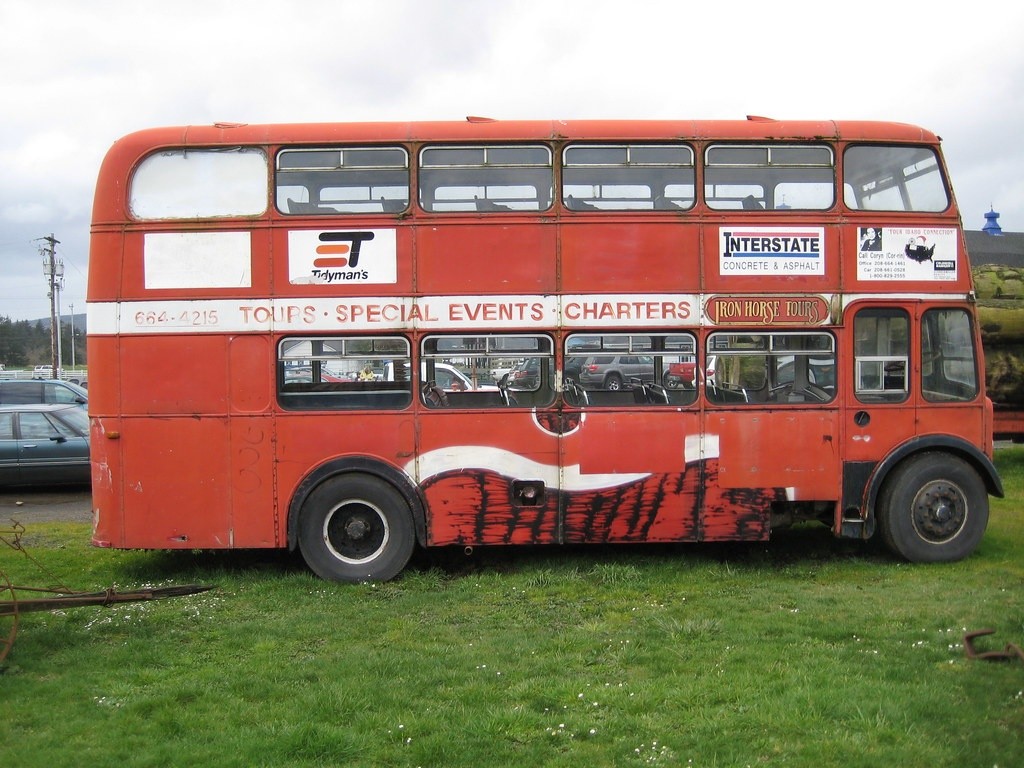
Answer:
[82,112,1006,583]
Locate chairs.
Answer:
[422,377,752,408]
[287,194,764,214]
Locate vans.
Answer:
[35,366,63,373]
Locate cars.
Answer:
[285,340,714,391]
[0,404,92,489]
[0,380,87,404]
